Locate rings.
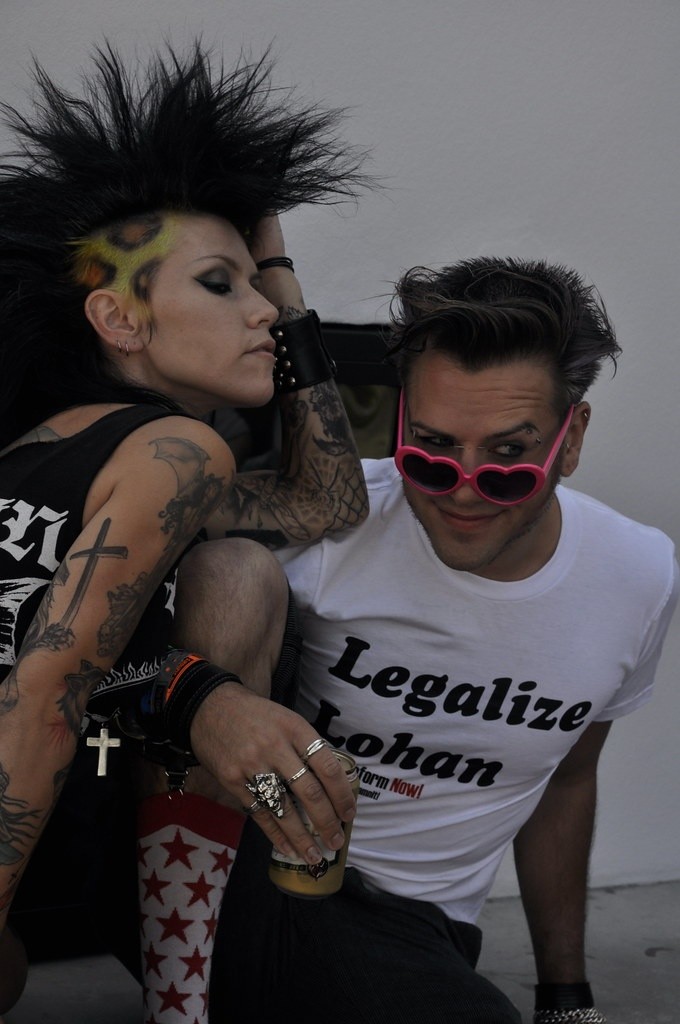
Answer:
[244,739,327,818]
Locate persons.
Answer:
[0,35,371,1024]
[86,260,679,1024]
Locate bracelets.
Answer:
[256,257,295,275]
[534,981,606,1024]
[270,308,337,396]
[150,649,245,797]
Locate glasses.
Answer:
[395,383,574,507]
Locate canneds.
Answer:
[267,748,359,900]
